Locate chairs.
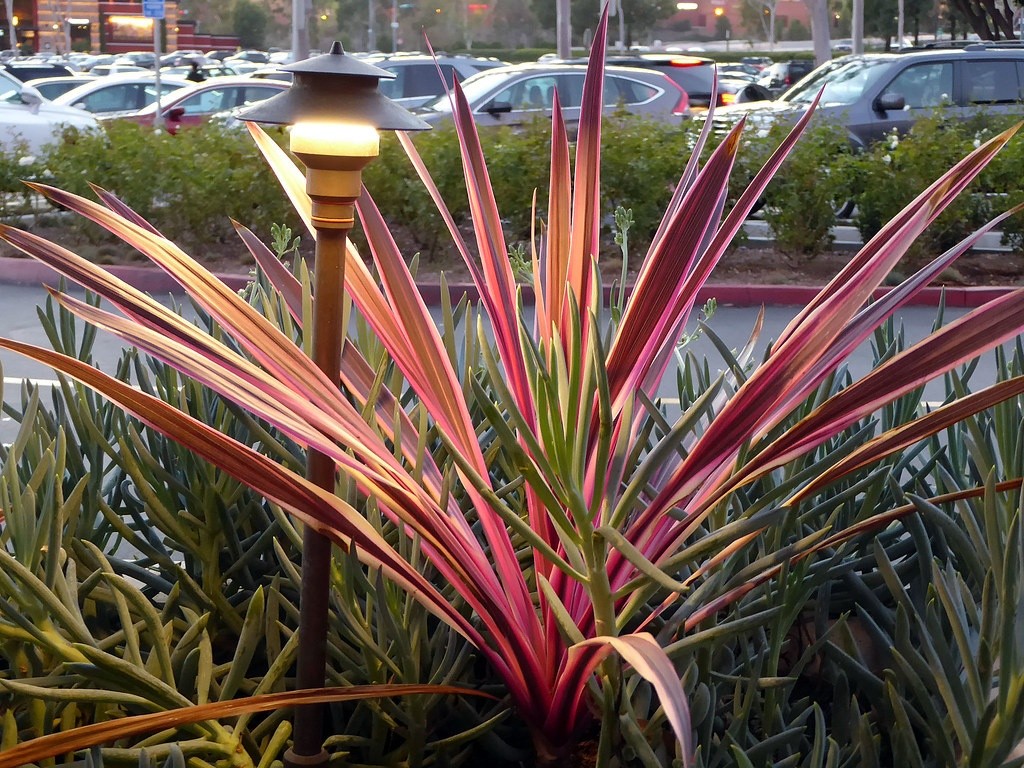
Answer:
[529,85,543,109]
[547,85,553,107]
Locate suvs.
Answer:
[599,57,736,118]
[370,57,514,113]
[688,38,1023,219]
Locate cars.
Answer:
[2,77,161,109]
[0,69,108,162]
[714,54,815,106]
[101,76,291,140]
[0,50,293,74]
[56,73,249,117]
[834,40,852,51]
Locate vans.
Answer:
[410,62,693,134]
